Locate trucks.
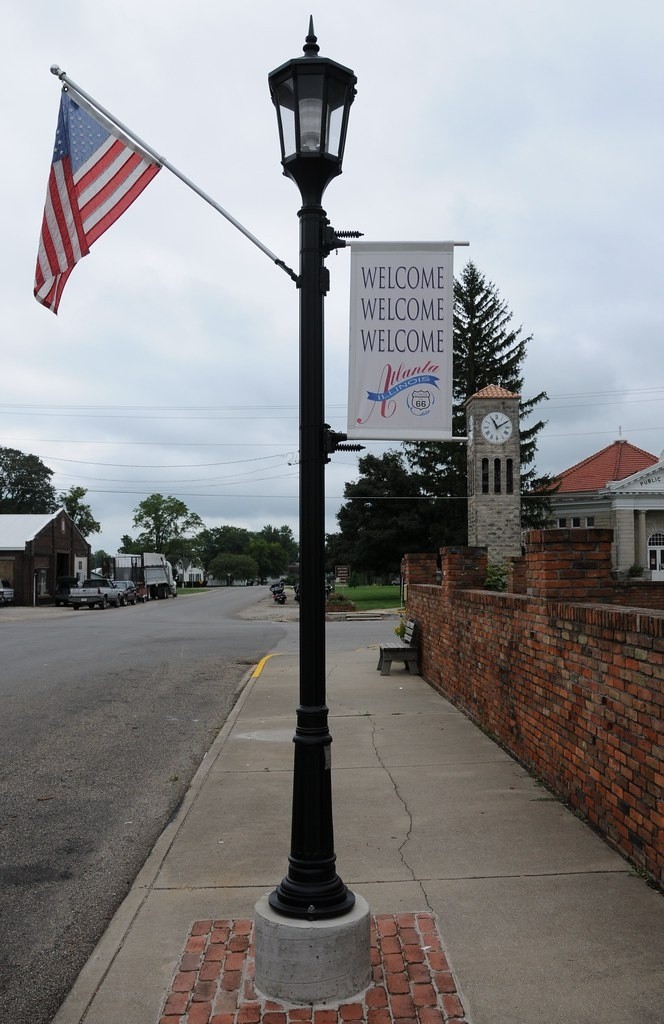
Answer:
[113,553,174,603]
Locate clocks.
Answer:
[481,412,513,444]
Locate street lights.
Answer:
[269,16,358,921]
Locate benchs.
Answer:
[377,618,421,676]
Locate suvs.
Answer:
[0,578,13,603]
[113,581,137,606]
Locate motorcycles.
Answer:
[269,582,287,605]
[294,584,300,602]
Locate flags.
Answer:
[32,84,161,313]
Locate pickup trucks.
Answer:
[68,577,123,612]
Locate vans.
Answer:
[55,577,82,606]
